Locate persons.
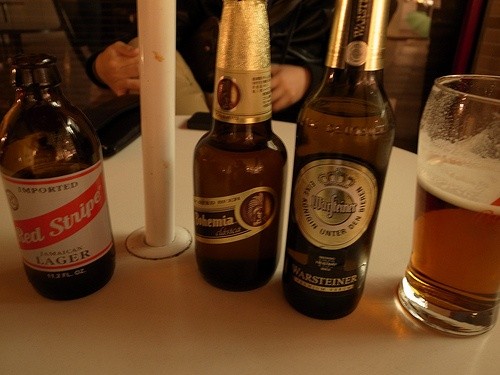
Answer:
[52,0,339,130]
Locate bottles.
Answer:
[1,54,116,302]
[281,0,398,322]
[194,0,289,291]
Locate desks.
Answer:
[1,114,500,375]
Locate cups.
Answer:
[395,73,499,338]
